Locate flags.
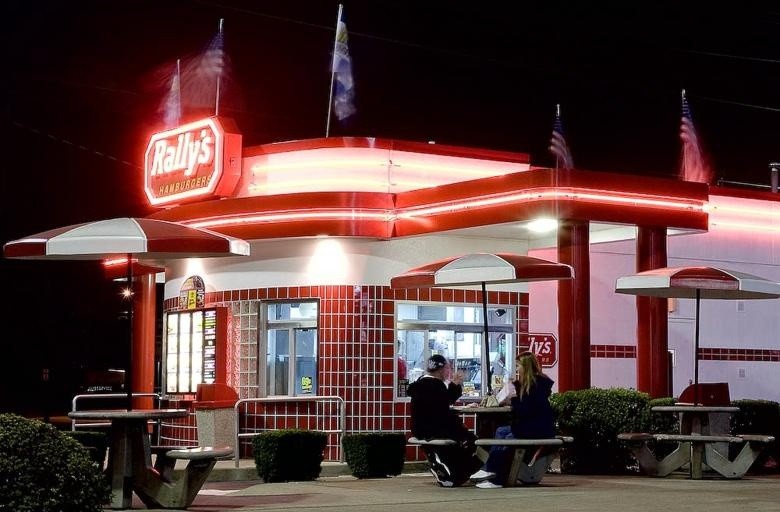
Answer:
[681,98,718,183]
[330,6,357,119]
[163,67,181,127]
[548,117,573,169]
[196,29,223,79]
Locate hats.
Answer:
[426,354,447,371]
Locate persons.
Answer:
[408,355,491,488]
[468,352,555,490]
[397,339,406,380]
[470,364,481,391]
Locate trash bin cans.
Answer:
[192,383,240,460]
[678,382,730,473]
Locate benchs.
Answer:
[139,445,234,511]
[406,436,574,487]
[616,433,775,478]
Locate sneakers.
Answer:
[430,466,452,486]
[469,470,495,482]
[427,453,450,477]
[476,480,502,489]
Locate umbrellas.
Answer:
[4,218,252,414]
[390,252,576,396]
[615,262,780,408]
